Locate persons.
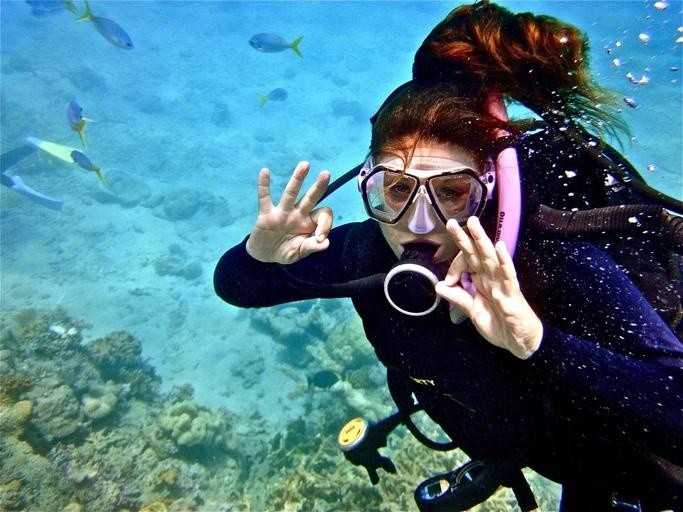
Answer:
[213,0,683,512]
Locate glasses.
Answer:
[357,155,496,227]
